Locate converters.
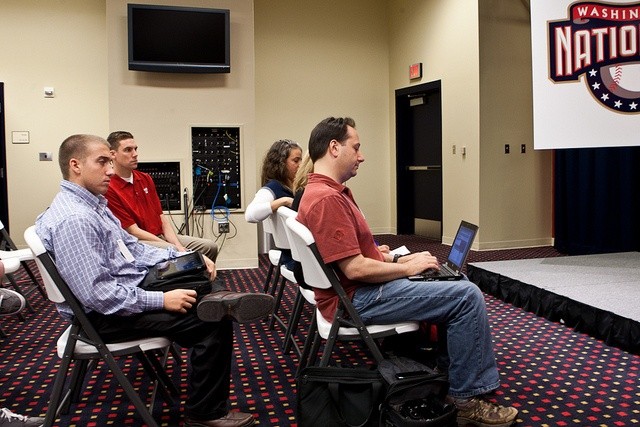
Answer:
[217,221,230,236]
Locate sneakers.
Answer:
[446,395,519,427]
[0,407,46,427]
[0,288,27,316]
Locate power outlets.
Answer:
[218,222,229,232]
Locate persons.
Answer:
[244,136,293,263]
[298,117,519,425]
[291,151,314,211]
[104,132,217,260]
[33,134,275,427]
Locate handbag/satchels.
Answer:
[139,250,213,299]
[296,366,389,427]
[377,352,459,427]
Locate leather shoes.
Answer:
[184,412,254,427]
[197,291,274,324]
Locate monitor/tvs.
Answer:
[128,3,230,74]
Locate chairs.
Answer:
[266,200,299,352]
[0,255,25,340]
[24,224,177,425]
[286,218,420,367]
[263,208,282,295]
[0,221,47,312]
[277,205,316,378]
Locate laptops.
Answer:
[408,220,479,281]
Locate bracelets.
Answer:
[393,252,402,263]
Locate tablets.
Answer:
[155,251,206,280]
